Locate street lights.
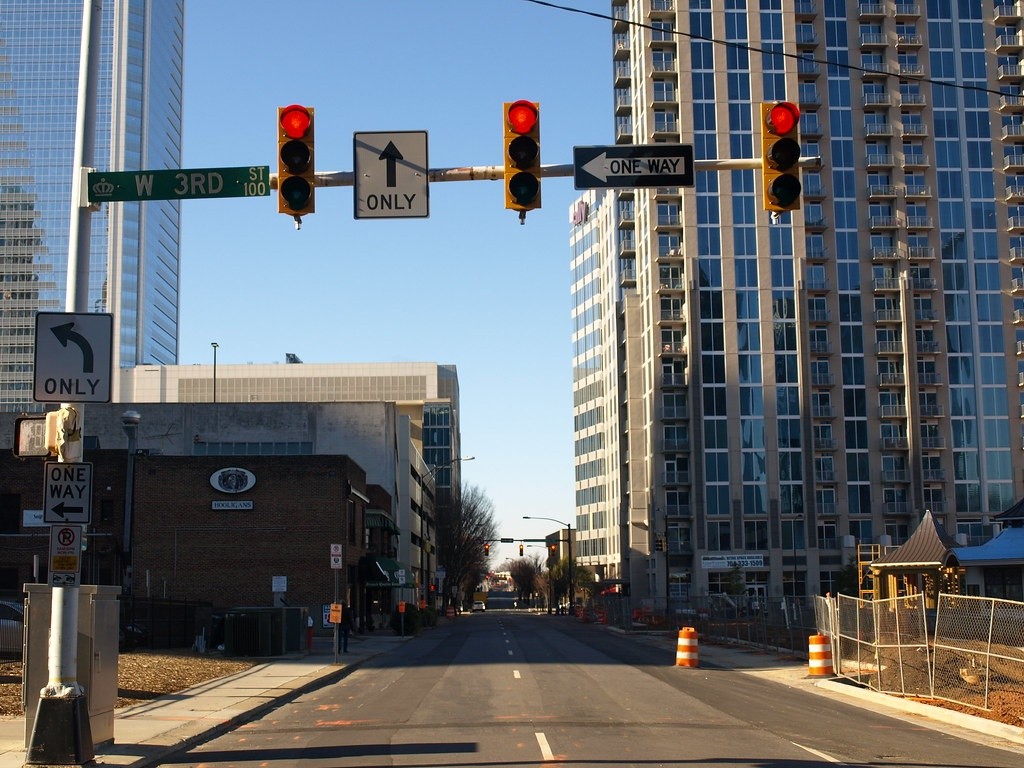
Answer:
[420,456,476,625]
[527,544,553,615]
[522,516,577,616]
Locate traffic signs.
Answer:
[572,141,695,194]
[42,459,94,525]
[354,128,430,220]
[32,309,114,405]
[88,164,272,204]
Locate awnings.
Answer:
[364,557,416,589]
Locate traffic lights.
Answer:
[520,545,524,556]
[759,100,802,214]
[276,102,315,220]
[503,98,542,216]
[552,544,556,556]
[485,544,489,556]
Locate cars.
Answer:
[471,601,486,612]
[118,616,152,652]
[0,599,26,654]
[118,599,153,624]
[133,598,216,649]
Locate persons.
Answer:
[337,598,352,654]
[513,601,518,609]
[556,605,565,615]
